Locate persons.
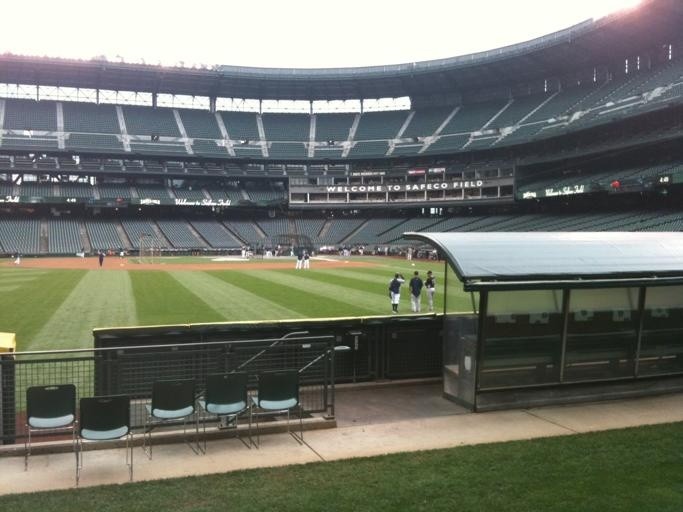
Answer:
[119,246,124,259]
[388,272,405,313]
[424,271,437,312]
[98,249,104,267]
[409,271,424,313]
[12,250,22,264]
[238,243,440,270]
[80,246,85,259]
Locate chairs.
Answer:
[24,369,303,486]
